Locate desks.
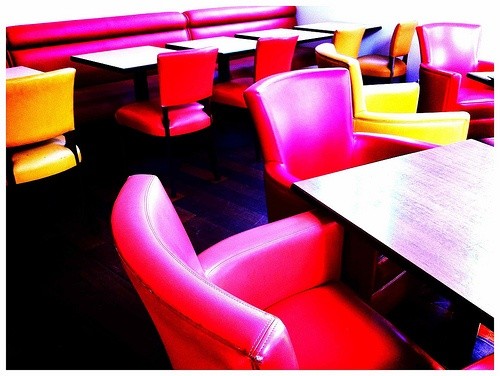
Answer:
[294,23,348,33]
[164,36,258,84]
[291,138,494,366]
[69,45,178,104]
[234,28,335,45]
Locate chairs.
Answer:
[7,23,494,370]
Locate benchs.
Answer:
[7,6,317,125]
